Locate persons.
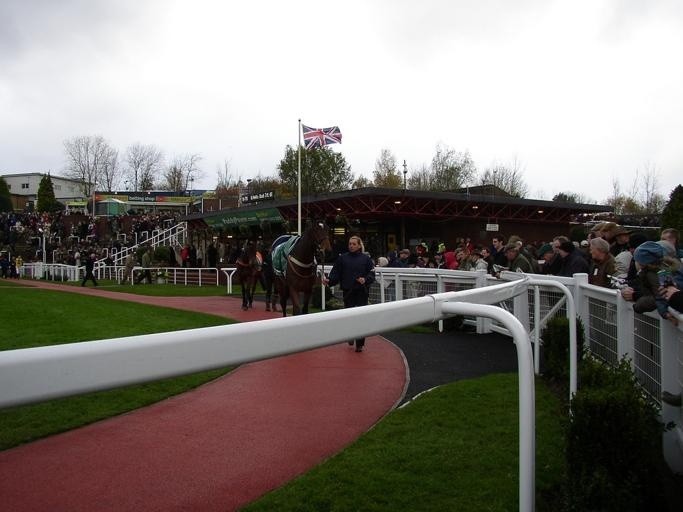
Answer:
[324,209,683,354]
[1,209,279,313]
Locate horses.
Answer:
[271,214,334,317]
[233,233,264,312]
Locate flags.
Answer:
[302,124,345,152]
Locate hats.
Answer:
[633,241,682,272]
[467,249,484,258]
[536,245,552,258]
[398,248,410,256]
[580,240,589,247]
[502,235,523,253]
[590,222,634,236]
